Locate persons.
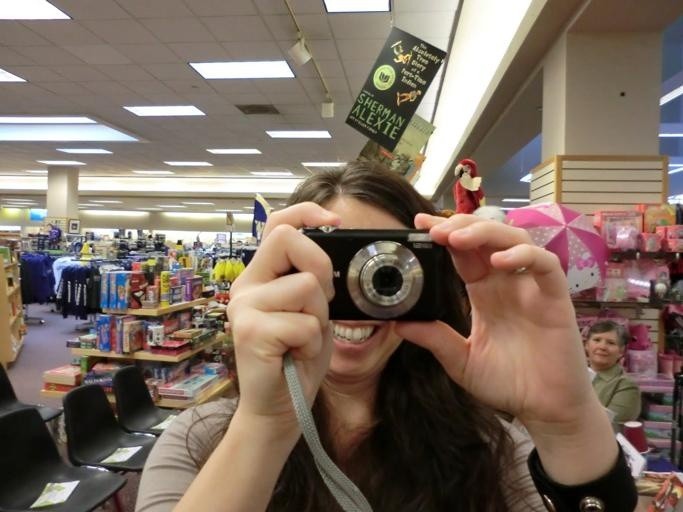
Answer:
[584,320,641,434]
[134,162,650,512]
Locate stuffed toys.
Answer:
[453,159,486,216]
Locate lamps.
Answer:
[321,89,335,120]
[288,32,314,66]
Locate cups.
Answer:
[623,421,649,455]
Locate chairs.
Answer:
[1,365,183,512]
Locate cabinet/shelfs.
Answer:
[38,296,236,411]
[0,252,26,369]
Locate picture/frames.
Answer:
[67,219,81,235]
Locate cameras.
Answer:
[284,226,440,323]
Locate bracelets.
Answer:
[527,442,637,511]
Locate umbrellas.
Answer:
[504,202,609,294]
